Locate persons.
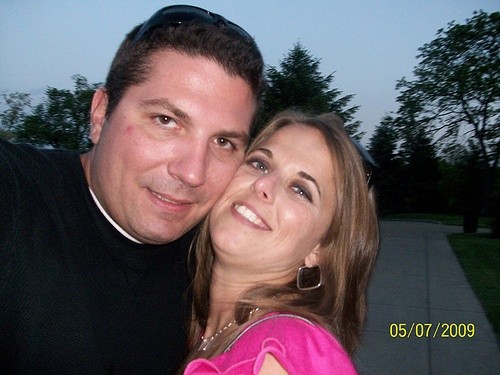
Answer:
[178,109,382,375]
[0,4,264,375]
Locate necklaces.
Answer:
[198,305,263,353]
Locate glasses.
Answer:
[348,131,378,196]
[128,5,256,70]
